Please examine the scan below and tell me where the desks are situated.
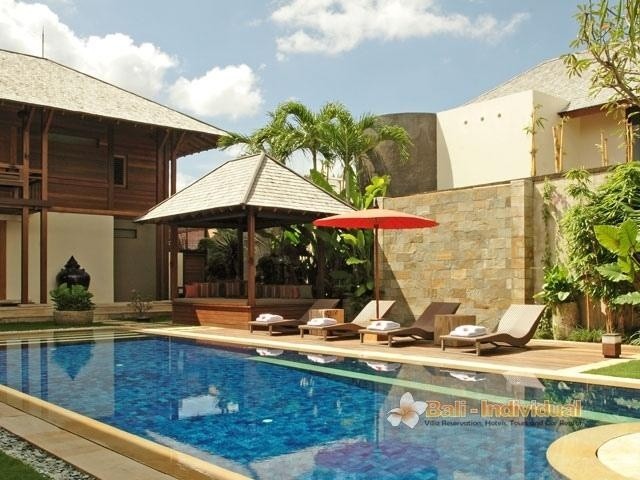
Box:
[546,418,640,480]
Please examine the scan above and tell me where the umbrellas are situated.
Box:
[313,206,440,320]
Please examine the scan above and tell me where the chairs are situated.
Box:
[247,296,547,354]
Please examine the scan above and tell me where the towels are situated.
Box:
[253,348,284,358]
[305,353,337,364]
[447,371,489,384]
[363,359,402,373]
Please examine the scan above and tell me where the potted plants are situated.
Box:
[536,263,579,340]
[558,167,640,360]
[48,283,96,324]
[125,288,154,322]
